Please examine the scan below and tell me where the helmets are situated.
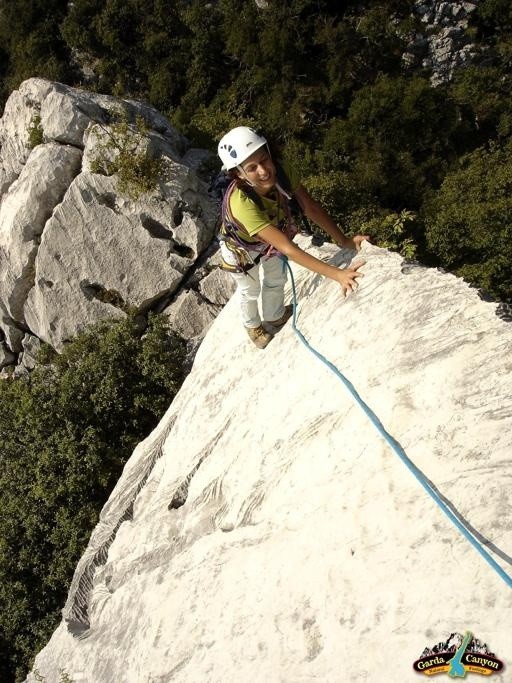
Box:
[218,126,267,172]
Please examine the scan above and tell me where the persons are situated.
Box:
[215,126,372,350]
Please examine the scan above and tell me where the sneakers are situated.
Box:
[246,323,273,349]
[267,305,293,328]
